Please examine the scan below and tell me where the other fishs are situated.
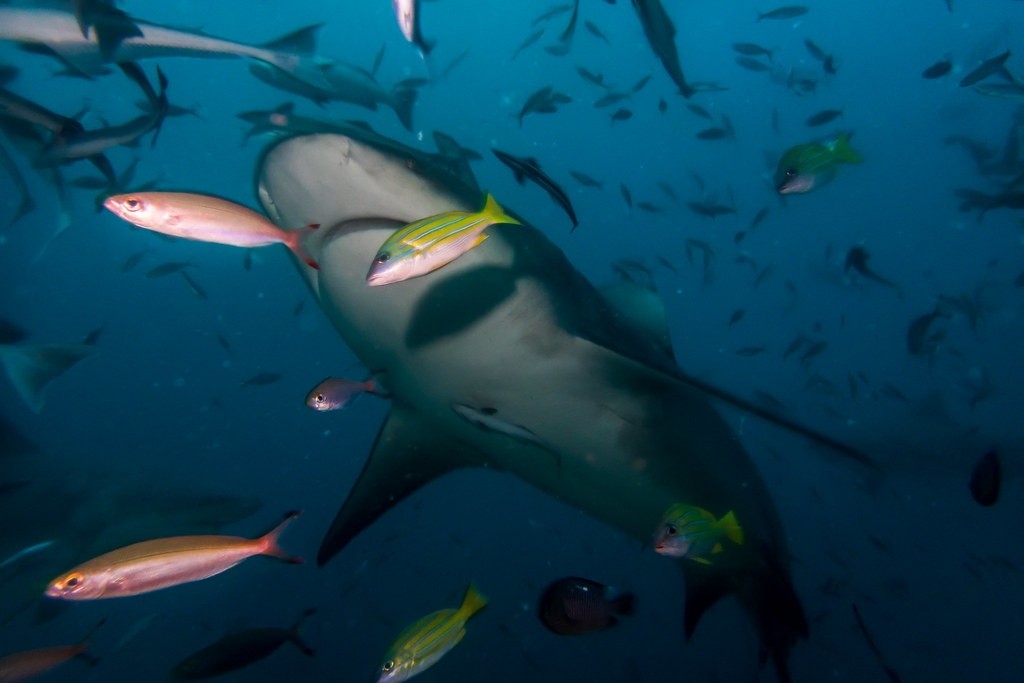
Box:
[0,0,1023,682]
